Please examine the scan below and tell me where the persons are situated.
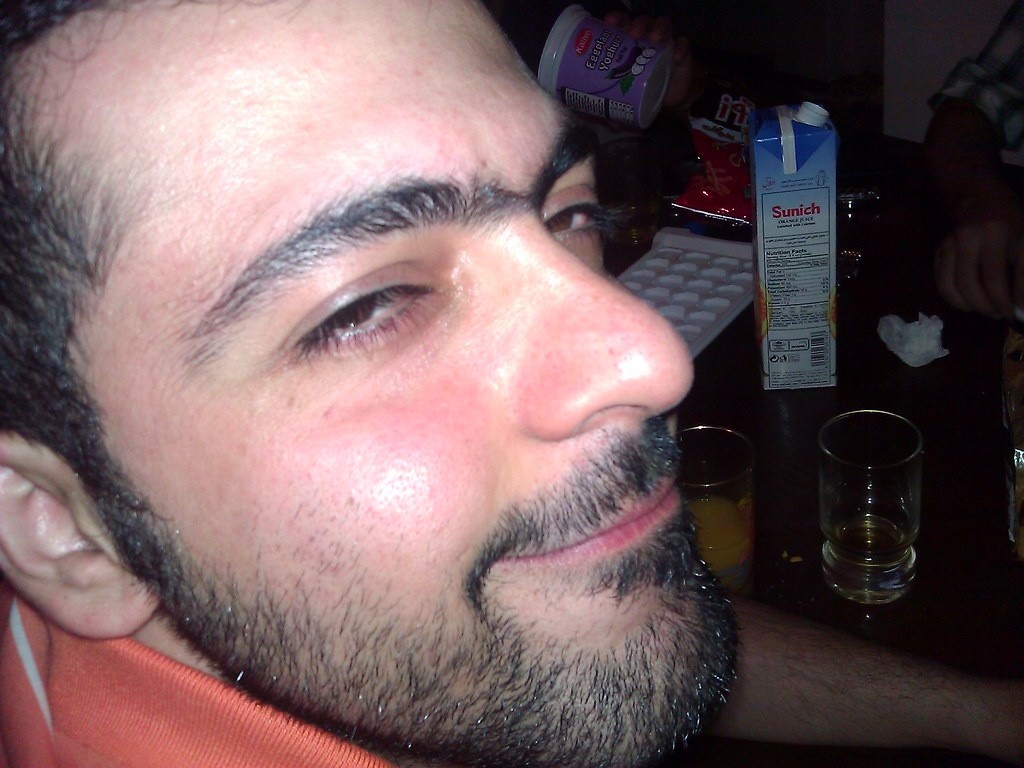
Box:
[483,0,707,131]
[920,0,1024,319]
[0,0,1024,768]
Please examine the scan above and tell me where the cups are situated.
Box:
[676,426,756,590]
[818,410,923,603]
[537,5,670,128]
[596,136,662,245]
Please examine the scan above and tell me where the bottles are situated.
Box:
[1002,304,1024,561]
